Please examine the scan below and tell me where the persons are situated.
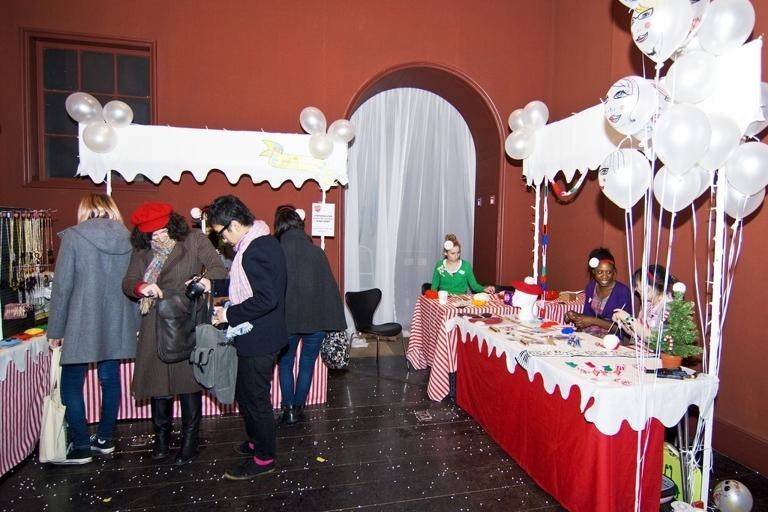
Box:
[47,191,142,465]
[273,206,347,425]
[612,265,673,353]
[430,234,496,296]
[565,248,633,341]
[184,194,291,480]
[121,199,216,466]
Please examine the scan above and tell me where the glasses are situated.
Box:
[216,226,229,238]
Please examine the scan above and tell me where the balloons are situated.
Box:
[103,99,133,129]
[603,76,658,137]
[724,179,766,221]
[65,92,104,125]
[699,0,755,56]
[299,106,326,136]
[654,165,701,213]
[744,82,768,137]
[327,119,355,143]
[507,108,524,131]
[309,133,334,160]
[504,128,536,160]
[82,121,117,153]
[713,479,753,512]
[697,112,741,173]
[724,142,768,195]
[631,0,692,64]
[522,100,550,130]
[652,103,712,175]
[598,147,652,210]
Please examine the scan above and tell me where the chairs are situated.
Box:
[495,283,516,294]
[420,281,434,296]
[342,287,410,384]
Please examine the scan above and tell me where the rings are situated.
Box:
[147,290,153,296]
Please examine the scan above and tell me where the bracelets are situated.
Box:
[624,315,635,325]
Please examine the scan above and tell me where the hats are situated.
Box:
[132,202,172,232]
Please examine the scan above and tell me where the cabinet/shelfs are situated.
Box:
[0,314,328,480]
[414,292,720,512]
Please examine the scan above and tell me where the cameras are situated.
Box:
[185,279,206,299]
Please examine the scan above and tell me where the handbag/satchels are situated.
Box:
[39,346,67,463]
[157,230,210,364]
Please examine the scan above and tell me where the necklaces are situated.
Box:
[0,210,54,294]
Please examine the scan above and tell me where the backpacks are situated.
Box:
[158,290,210,364]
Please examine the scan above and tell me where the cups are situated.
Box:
[438,291,447,305]
[602,334,619,350]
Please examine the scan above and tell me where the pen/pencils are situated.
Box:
[490,327,497,332]
[520,340,526,345]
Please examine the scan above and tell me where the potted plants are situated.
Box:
[640,296,702,371]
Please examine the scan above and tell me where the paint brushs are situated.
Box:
[646,366,701,379]
[608,304,627,333]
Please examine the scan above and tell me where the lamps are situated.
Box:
[489,195,497,206]
[476,197,483,209]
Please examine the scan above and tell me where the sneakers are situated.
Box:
[225,457,274,480]
[55,442,92,464]
[90,434,115,454]
[235,441,255,456]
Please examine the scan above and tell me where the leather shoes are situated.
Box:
[151,443,171,460]
[175,442,198,466]
[283,404,299,423]
